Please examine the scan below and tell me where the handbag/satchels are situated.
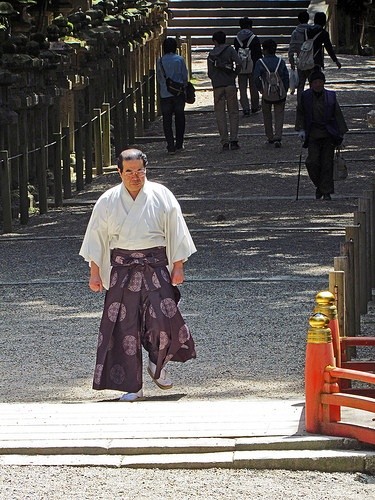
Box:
[333,146,348,180]
[289,66,298,95]
[166,78,186,98]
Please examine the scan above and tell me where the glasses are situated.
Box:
[119,168,148,178]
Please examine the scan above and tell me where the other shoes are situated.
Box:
[168,146,182,155]
[223,144,229,150]
[231,141,240,150]
[251,108,262,115]
[268,140,281,148]
[243,112,250,117]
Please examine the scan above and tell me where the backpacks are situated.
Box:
[236,34,256,74]
[258,57,287,102]
[297,29,324,71]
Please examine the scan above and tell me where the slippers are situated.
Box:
[147,366,174,390]
[117,397,146,402]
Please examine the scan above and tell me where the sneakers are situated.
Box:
[316,187,323,199]
[324,193,331,201]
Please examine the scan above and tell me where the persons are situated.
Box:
[253,39,290,147]
[207,31,242,148]
[296,72,348,199]
[288,12,311,70]
[79,149,197,401]
[156,39,195,151]
[296,12,342,104]
[234,17,261,116]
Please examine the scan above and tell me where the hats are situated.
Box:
[307,71,326,83]
